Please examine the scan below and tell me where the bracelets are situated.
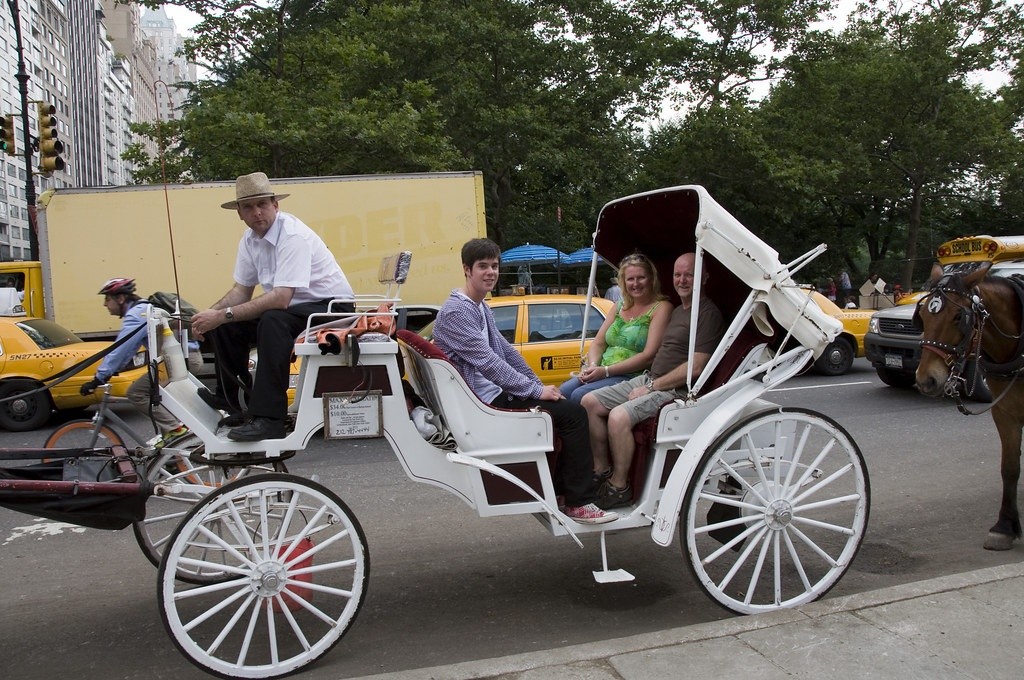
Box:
[605,366,611,379]
[647,380,655,392]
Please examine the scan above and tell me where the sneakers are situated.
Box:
[588,464,615,493]
[154,423,190,449]
[157,465,178,477]
[557,495,566,510]
[594,479,634,510]
[197,387,235,416]
[227,417,286,442]
[564,502,619,525]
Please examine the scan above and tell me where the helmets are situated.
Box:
[97,278,137,296]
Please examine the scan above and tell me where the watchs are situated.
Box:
[225,306,234,323]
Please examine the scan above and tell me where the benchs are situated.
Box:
[633,326,773,451]
[294,250,414,356]
[395,328,554,458]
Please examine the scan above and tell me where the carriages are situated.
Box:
[0,179,872,680]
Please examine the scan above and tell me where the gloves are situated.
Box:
[79,376,103,396]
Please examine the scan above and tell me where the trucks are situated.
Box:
[863,232,1024,402]
[0,170,485,342]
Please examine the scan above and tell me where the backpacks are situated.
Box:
[130,291,201,326]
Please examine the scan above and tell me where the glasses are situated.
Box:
[621,254,652,273]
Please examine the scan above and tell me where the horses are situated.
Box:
[912,261,1024,551]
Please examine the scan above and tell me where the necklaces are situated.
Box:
[629,301,650,323]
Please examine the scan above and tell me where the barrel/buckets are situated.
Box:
[251,503,313,612]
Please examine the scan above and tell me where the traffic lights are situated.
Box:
[38,102,64,171]
[0,115,15,155]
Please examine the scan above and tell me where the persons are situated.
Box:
[558,253,727,511]
[870,273,878,284]
[80,277,203,449]
[825,278,836,304]
[192,172,356,442]
[837,272,851,309]
[7,278,16,287]
[812,279,824,294]
[432,238,618,525]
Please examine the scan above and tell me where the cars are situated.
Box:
[896,275,932,305]
[796,287,879,376]
[397,295,616,391]
[0,317,170,432]
[242,304,442,416]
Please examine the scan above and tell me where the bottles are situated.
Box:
[161,327,188,381]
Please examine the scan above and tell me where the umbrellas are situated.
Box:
[499,242,569,294]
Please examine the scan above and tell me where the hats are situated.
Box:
[826,278,833,282]
[609,277,618,284]
[220,172,290,210]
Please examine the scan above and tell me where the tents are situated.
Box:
[553,248,603,293]
